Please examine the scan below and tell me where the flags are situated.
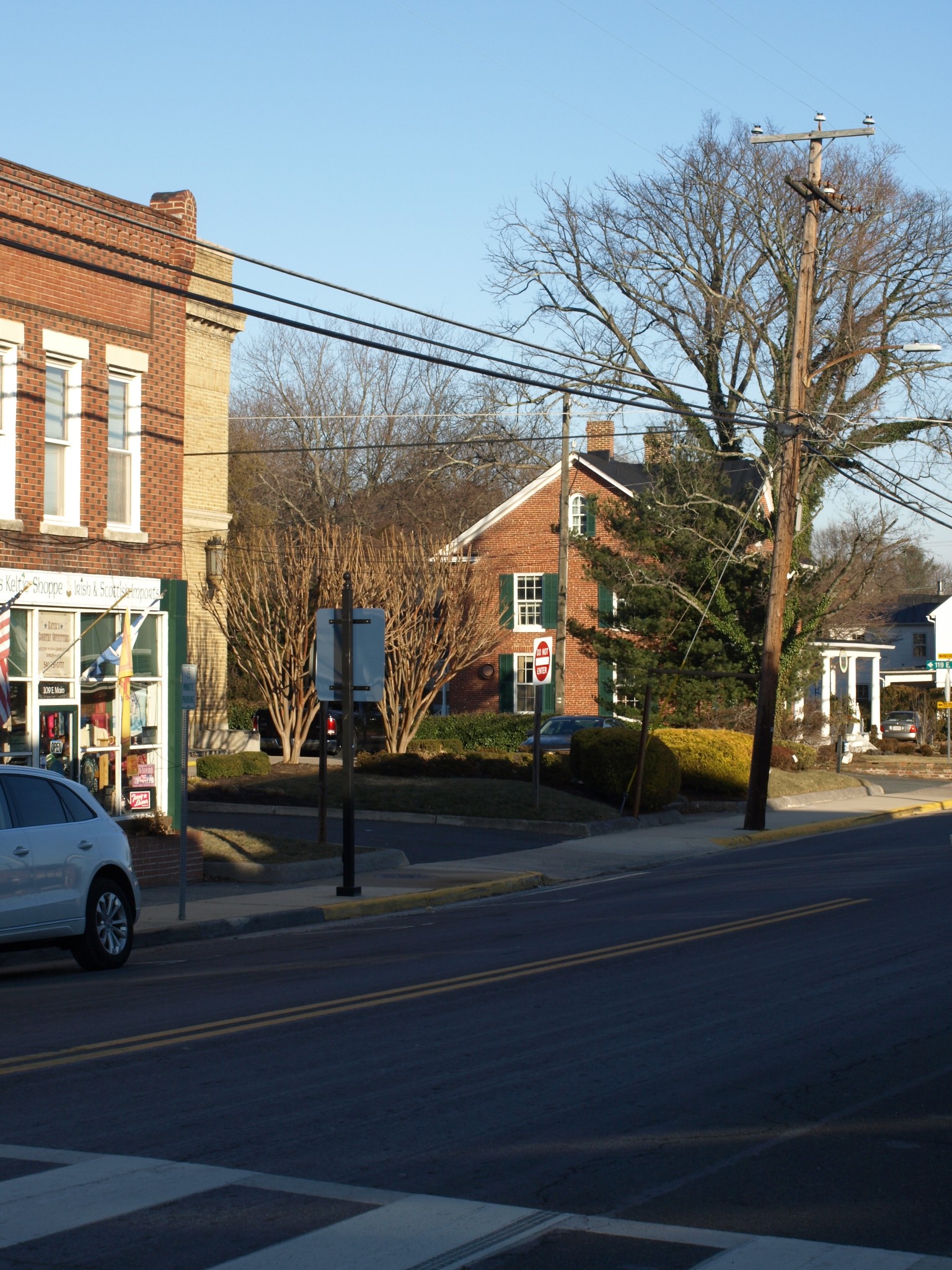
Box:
[0,589,150,754]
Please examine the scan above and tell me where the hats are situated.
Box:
[81,717,90,728]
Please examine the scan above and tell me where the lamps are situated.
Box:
[207,534,225,592]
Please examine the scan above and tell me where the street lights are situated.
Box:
[740,339,944,831]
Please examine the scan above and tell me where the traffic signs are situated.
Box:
[937,702,952,709]
[926,660,952,669]
[938,654,952,659]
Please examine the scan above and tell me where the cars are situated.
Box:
[880,710,923,746]
[0,764,142,972]
[253,685,388,756]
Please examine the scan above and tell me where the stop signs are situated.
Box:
[535,641,550,682]
